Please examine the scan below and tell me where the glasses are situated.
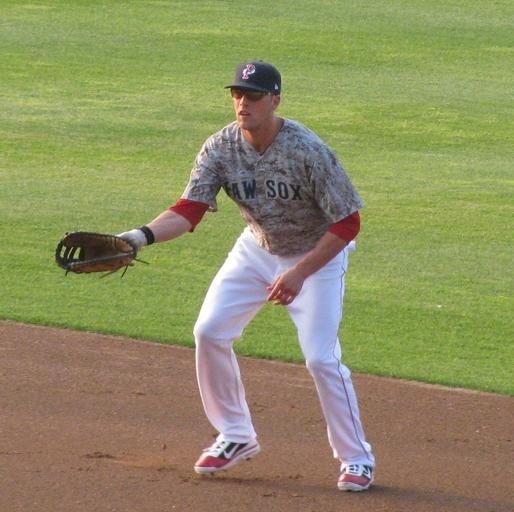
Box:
[231,86,270,101]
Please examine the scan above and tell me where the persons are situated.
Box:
[114,57,377,493]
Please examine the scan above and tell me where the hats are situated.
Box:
[224,60,282,95]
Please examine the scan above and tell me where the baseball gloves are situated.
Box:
[56,232,136,274]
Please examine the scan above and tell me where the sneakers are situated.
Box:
[337,463,374,492]
[193,436,261,476]
